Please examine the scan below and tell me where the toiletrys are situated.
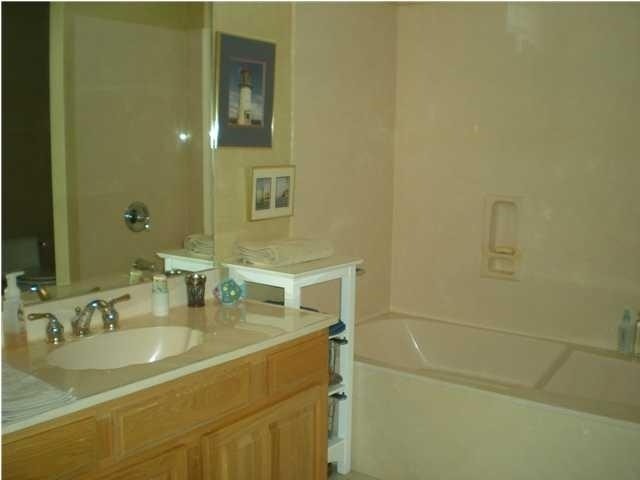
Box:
[3,270,29,349]
[149,271,171,318]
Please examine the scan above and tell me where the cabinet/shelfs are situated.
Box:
[221,251,364,474]
[155,249,213,276]
[1,327,329,480]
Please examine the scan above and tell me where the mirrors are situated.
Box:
[2,1,213,312]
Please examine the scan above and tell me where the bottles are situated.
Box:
[151,274,169,316]
[618,310,640,356]
[129,270,143,285]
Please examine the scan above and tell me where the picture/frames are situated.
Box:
[215,29,277,149]
[249,164,296,221]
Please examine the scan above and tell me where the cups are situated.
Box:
[185,277,206,306]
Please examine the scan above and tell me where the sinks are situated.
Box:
[44,321,209,372]
[21,284,98,304]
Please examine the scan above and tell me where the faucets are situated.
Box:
[29,283,49,299]
[69,296,119,336]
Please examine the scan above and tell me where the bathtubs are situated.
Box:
[353,311,639,479]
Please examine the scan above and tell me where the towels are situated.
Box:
[240,239,334,267]
[184,234,214,260]
[1,362,78,421]
